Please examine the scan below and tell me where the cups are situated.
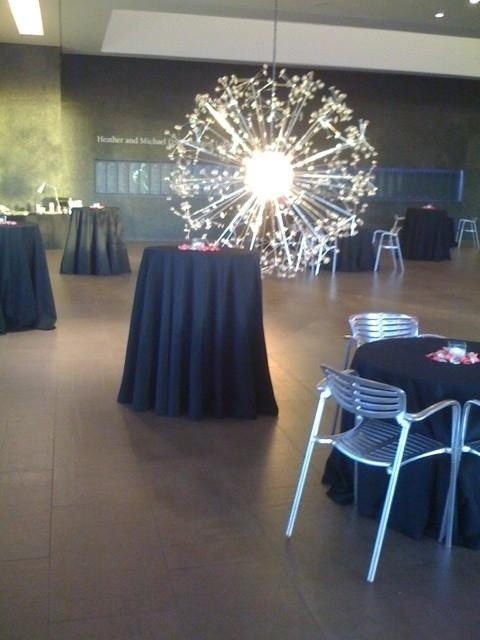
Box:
[1,215,6,224]
[448,341,467,362]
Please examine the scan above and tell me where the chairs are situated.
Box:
[437,399,480,546]
[329,311,446,438]
[286,363,460,583]
[456,216,479,250]
[370,216,406,275]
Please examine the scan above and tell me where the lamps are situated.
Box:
[37,183,62,212]
[162,0,378,280]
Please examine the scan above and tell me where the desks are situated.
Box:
[118,246,278,420]
[0,211,72,250]
[397,206,456,263]
[0,219,57,335]
[321,338,480,552]
[306,222,379,272]
[60,208,131,276]
